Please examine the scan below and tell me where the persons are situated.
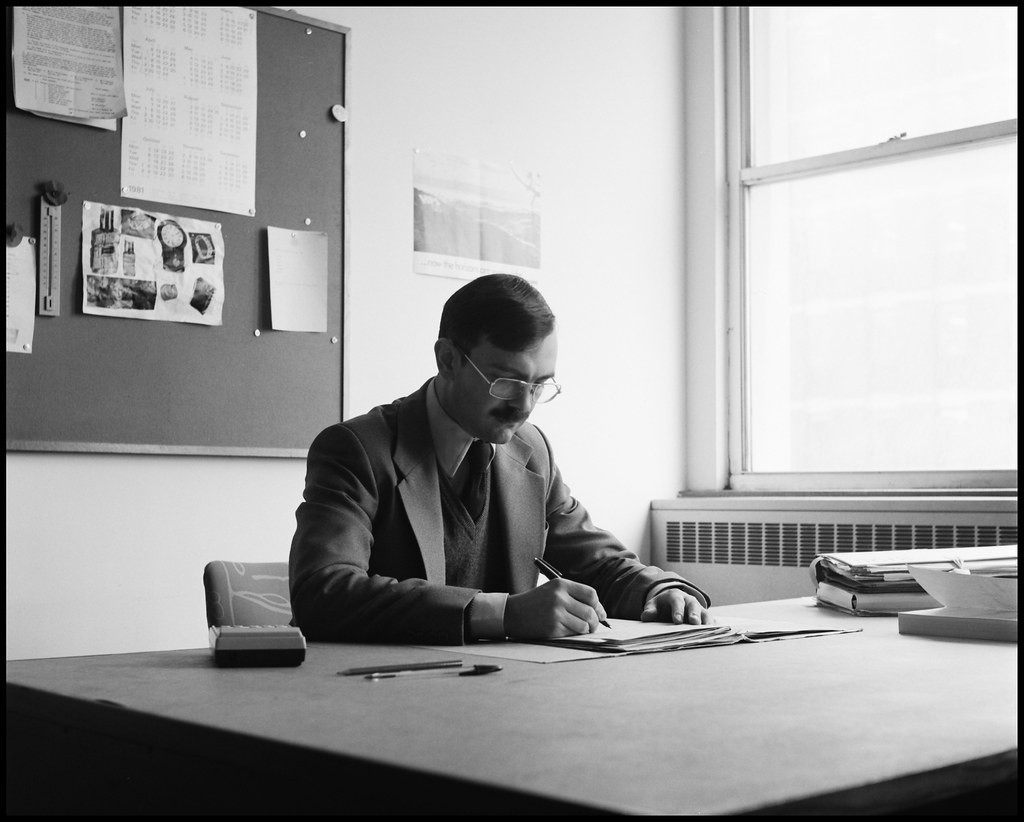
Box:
[288,274,712,641]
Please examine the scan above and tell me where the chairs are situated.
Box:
[205,561,294,628]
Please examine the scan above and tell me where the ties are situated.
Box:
[459,440,494,525]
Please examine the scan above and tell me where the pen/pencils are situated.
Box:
[335,659,503,680]
[532,558,612,630]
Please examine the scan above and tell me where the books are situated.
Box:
[897,563,1017,644]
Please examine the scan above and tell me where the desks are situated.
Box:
[7,595,1022,820]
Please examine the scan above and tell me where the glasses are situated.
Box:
[462,352,563,406]
